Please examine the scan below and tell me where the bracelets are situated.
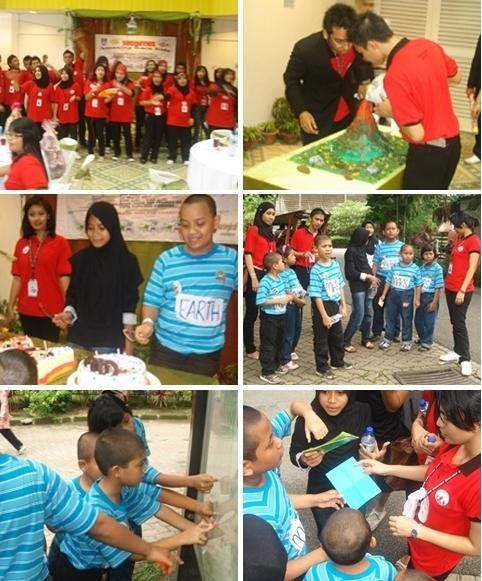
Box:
[142,317,155,326]
[458,289,467,296]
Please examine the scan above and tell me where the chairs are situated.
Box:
[462,154,480,164]
[315,369,336,380]
[364,505,388,532]
[439,350,461,362]
[330,362,352,370]
[259,374,281,384]
[284,361,299,370]
[289,351,298,360]
[459,359,473,376]
[365,335,431,352]
[77,140,193,166]
[342,344,357,353]
[246,350,262,361]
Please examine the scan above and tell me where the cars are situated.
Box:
[207,129,238,155]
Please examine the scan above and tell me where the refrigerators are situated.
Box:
[273,365,289,375]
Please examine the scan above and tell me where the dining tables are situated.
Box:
[243,97,301,152]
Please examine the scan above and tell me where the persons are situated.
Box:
[344,220,446,355]
[245,199,279,362]
[276,245,308,373]
[282,2,377,147]
[289,207,329,290]
[348,9,462,190]
[1,453,186,581]
[1,349,39,386]
[43,391,223,581]
[350,1,376,82]
[255,253,293,385]
[437,210,480,379]
[306,232,353,382]
[0,28,237,165]
[134,193,238,378]
[1,390,27,457]
[7,197,73,344]
[1,119,51,190]
[463,33,480,166]
[242,390,480,581]
[53,202,145,358]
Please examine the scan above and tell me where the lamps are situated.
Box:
[60,138,78,152]
[70,154,96,189]
[146,167,189,190]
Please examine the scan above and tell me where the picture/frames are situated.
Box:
[1,330,222,385]
[243,123,408,189]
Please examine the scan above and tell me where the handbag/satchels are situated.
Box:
[458,289,467,296]
[142,317,155,326]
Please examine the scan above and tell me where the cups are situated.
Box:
[6,131,23,139]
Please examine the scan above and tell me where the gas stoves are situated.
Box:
[1,335,151,386]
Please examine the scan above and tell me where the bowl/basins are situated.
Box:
[333,50,347,77]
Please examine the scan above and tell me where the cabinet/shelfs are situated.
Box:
[418,396,430,415]
[329,312,342,327]
[360,427,376,454]
[280,289,309,300]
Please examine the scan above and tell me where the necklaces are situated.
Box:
[333,50,347,77]
